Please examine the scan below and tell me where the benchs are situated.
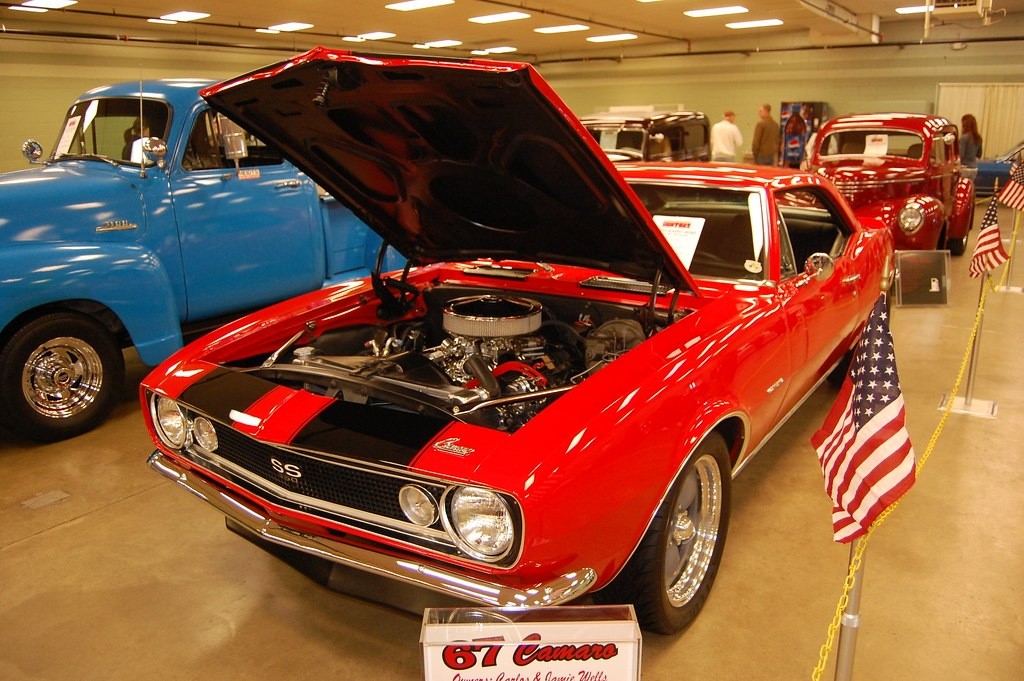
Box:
[652,209,840,272]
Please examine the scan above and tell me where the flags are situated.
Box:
[997,154,1024,211]
[809,290,916,544]
[968,193,1011,279]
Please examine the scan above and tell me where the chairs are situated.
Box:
[842,142,864,153]
[908,144,923,158]
[648,135,671,154]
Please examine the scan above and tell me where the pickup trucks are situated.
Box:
[0,80,412,443]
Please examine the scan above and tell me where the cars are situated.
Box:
[974,140,1024,200]
[579,111,711,172]
[805,113,975,263]
[138,44,896,635]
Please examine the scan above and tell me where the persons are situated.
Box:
[711,111,742,162]
[960,115,983,182]
[752,105,778,165]
[122,117,150,160]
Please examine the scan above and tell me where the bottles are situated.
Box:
[784,104,807,170]
[802,106,813,139]
